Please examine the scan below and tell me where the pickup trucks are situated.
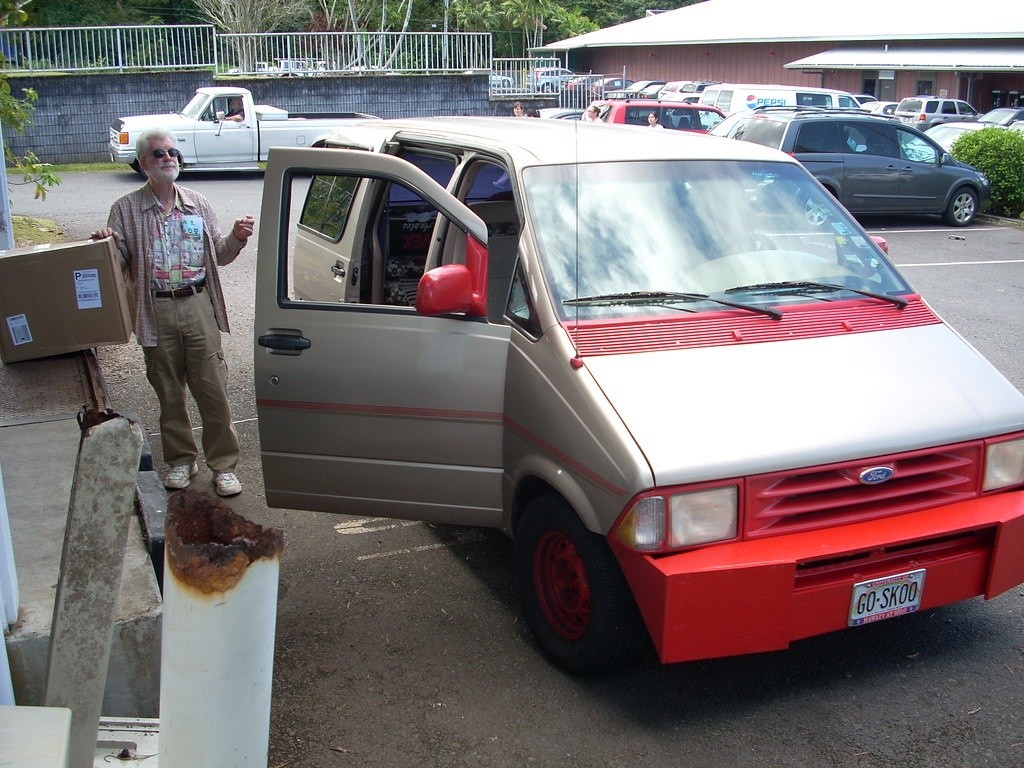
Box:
[105,86,386,177]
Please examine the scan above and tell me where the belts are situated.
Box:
[151,280,207,297]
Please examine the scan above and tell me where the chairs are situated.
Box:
[382,201,530,325]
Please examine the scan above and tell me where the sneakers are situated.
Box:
[164,460,197,489]
[211,472,242,496]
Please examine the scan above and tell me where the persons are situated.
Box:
[694,180,777,260]
[588,106,603,123]
[225,97,244,122]
[524,109,540,117]
[513,102,524,117]
[648,112,663,128]
[90,129,255,496]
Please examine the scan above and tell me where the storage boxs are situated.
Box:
[0,235,133,364]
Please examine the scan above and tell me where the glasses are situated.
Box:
[144,147,181,156]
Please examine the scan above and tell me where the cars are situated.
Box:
[457,63,1024,226]
[253,110,1024,685]
